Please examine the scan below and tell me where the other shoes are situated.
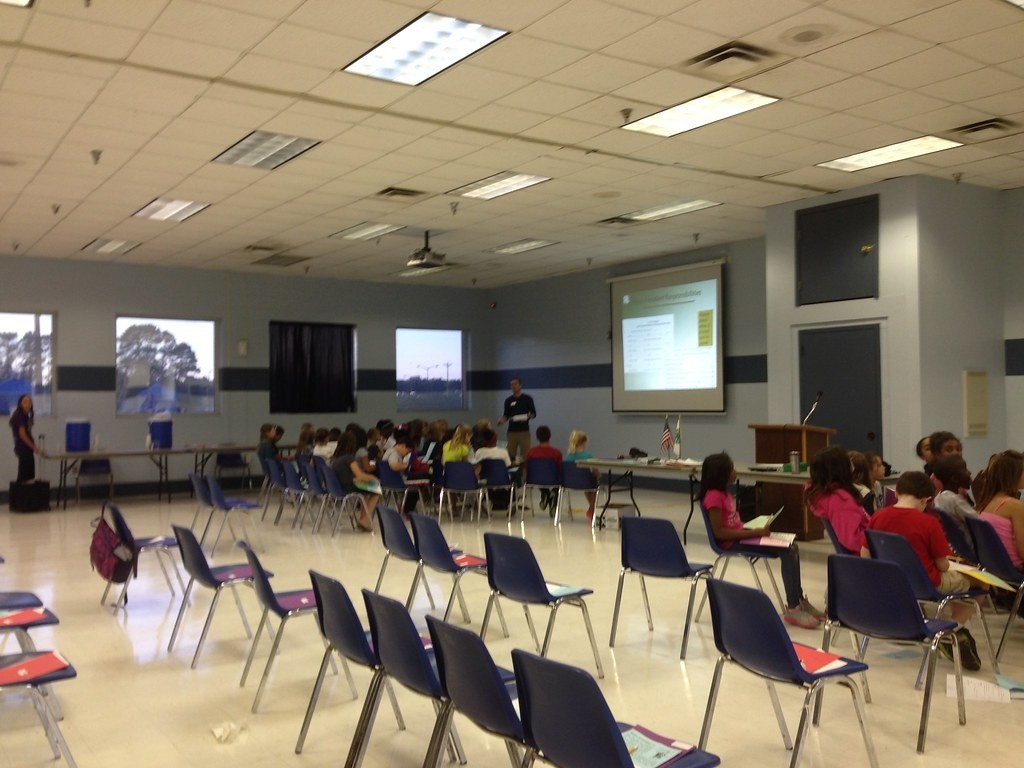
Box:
[586,510,593,519]
[539,493,550,510]
[549,498,558,517]
[358,522,372,532]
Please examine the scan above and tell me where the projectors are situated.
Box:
[407,252,446,267]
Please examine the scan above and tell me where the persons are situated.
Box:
[9,395,42,485]
[804,443,885,559]
[693,453,825,628]
[565,430,601,519]
[914,431,979,556]
[976,449,1024,562]
[861,470,989,671]
[258,419,519,532]
[525,426,563,517]
[497,379,536,459]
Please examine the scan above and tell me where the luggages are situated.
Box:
[10,434,52,514]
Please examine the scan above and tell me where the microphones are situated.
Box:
[815,390,823,402]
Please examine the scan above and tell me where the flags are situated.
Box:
[673,420,680,456]
[661,421,673,453]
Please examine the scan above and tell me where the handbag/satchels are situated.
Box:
[88,519,132,585]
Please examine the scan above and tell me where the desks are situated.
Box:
[574,458,906,546]
[34,443,304,511]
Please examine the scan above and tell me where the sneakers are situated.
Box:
[783,605,821,627]
[798,596,826,620]
[955,628,982,671]
[937,638,954,660]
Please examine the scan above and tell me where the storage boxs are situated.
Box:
[148,415,174,449]
[594,504,635,530]
[66,419,91,451]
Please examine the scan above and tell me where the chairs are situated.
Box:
[0,441,1024,768]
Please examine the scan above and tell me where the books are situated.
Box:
[740,532,797,548]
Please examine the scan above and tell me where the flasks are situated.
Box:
[789,451,801,475]
